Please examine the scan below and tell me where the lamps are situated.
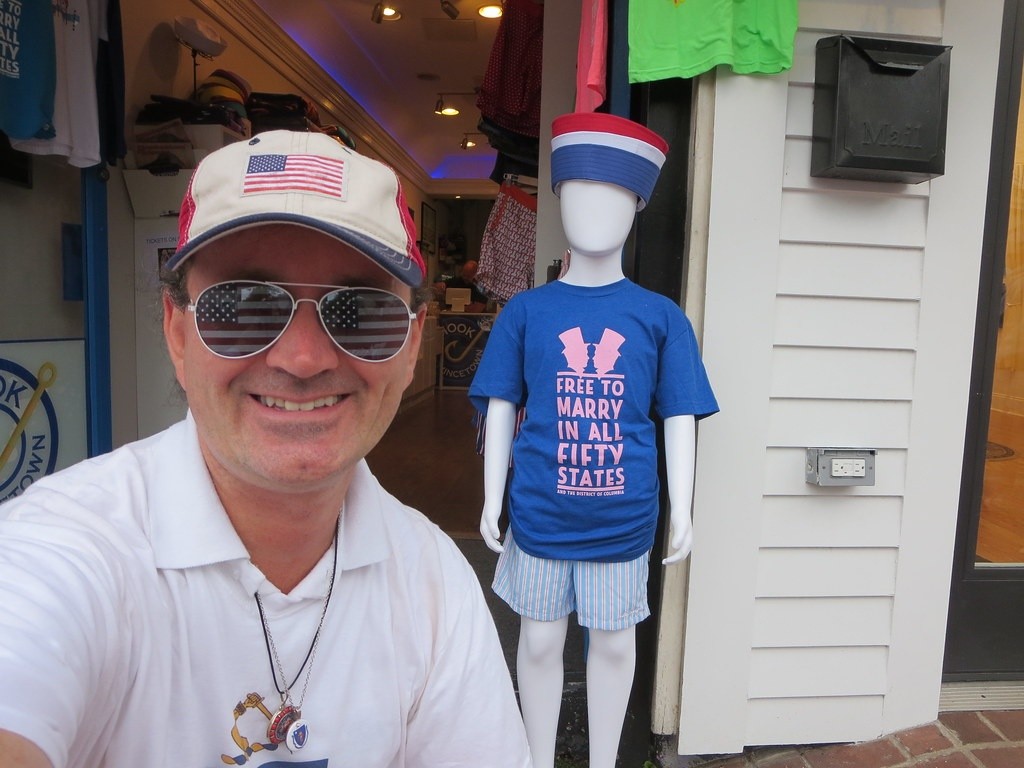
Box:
[371,0,385,25]
[439,0,458,20]
[434,95,444,115]
[462,133,470,149]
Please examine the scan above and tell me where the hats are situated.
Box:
[197,67,253,120]
[173,16,227,57]
[162,128,429,289]
[551,112,671,212]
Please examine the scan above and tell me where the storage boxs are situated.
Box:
[120,96,253,216]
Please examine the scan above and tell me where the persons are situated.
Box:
[0,128,536,768]
[431,258,490,314]
[467,114,720,768]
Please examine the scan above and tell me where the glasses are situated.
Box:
[184,278,419,364]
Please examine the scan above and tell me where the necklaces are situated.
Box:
[235,517,342,759]
